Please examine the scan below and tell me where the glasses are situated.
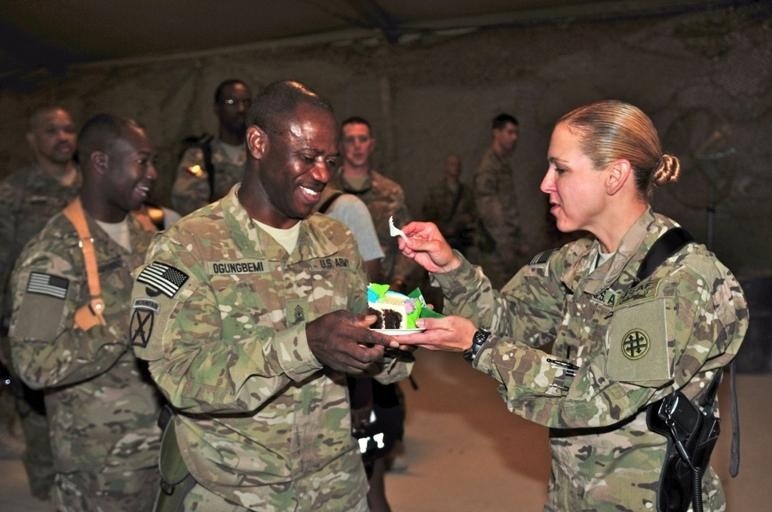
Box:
[221,96,251,107]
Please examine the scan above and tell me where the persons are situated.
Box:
[1,79,416,512]
[386,98,751,512]
[472,113,530,289]
[419,154,476,260]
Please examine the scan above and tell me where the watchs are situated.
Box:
[463,329,491,361]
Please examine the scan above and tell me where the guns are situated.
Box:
[658,390,721,476]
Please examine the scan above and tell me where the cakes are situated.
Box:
[367,302,405,329]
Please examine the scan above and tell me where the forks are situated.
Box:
[388,215,410,242]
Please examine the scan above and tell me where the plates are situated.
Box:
[365,326,425,338]
